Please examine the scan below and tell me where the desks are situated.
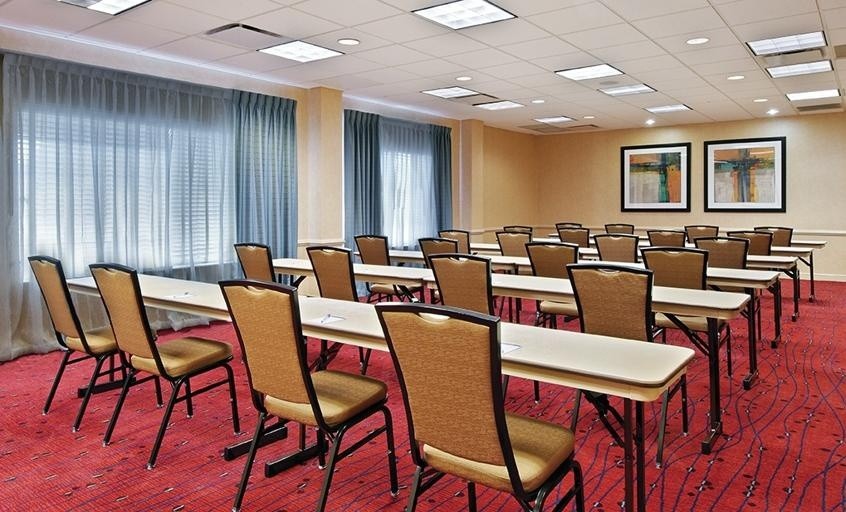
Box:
[63,271,696,512]
[353,249,781,391]
[272,257,752,456]
[533,237,813,300]
[547,233,829,302]
[461,242,803,320]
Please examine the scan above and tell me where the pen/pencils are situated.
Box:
[321,312,330,324]
[173,292,188,299]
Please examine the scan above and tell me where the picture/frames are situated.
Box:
[620,142,691,213]
[704,136,787,213]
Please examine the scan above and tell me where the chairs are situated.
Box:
[428,254,540,404]
[496,231,532,256]
[418,237,460,305]
[726,231,773,256]
[27,255,158,433]
[593,234,642,263]
[504,225,533,231]
[88,263,241,468]
[525,242,580,329]
[685,225,719,244]
[640,247,734,380]
[374,302,585,512]
[754,226,794,247]
[555,222,582,230]
[566,263,689,467]
[438,229,471,254]
[693,236,762,342]
[558,227,590,248]
[647,230,686,248]
[605,223,634,234]
[234,242,276,365]
[354,235,427,305]
[218,278,399,512]
[306,246,364,371]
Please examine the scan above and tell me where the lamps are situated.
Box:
[338,38,361,47]
[642,103,694,115]
[58,1,155,17]
[410,0,519,31]
[764,59,835,80]
[554,63,625,82]
[785,88,842,102]
[455,76,473,82]
[472,99,526,111]
[257,39,346,63]
[421,86,481,99]
[745,30,829,57]
[686,36,710,46]
[597,82,658,97]
